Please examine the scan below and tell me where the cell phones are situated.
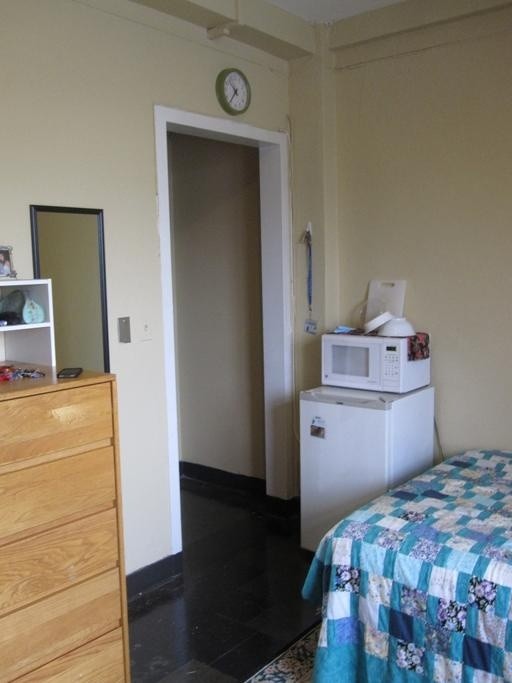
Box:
[57,368,82,379]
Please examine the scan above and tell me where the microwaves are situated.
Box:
[321,334,431,393]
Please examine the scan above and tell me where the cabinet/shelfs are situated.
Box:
[1,279,57,364]
[0,360,129,681]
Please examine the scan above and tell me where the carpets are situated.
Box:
[245,621,321,682]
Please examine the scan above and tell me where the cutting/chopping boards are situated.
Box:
[365,279,406,322]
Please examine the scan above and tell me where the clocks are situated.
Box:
[216,68,251,114]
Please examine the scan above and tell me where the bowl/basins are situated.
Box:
[378,318,416,336]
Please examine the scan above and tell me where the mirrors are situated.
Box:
[26,204,111,372]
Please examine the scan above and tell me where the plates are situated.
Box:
[363,311,391,336]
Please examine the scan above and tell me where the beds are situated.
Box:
[301,446,510,676]
